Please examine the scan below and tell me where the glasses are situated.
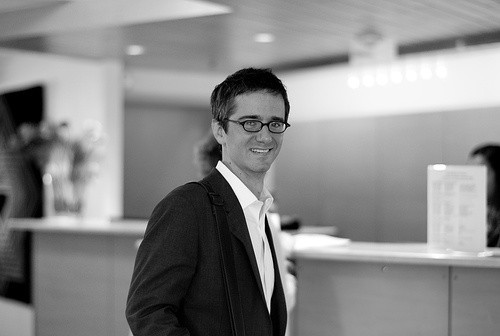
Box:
[226,119,290,134]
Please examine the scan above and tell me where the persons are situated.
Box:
[126,68,291,336]
[195,129,221,180]
[467,144,500,248]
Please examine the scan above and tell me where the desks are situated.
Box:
[10,220,499,336]
